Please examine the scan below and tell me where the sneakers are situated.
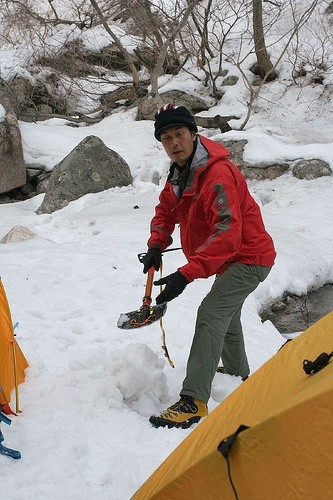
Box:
[217,367,248,382]
[149,396,208,429]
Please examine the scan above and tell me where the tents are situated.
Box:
[125,310,333,500]
[0,277,30,405]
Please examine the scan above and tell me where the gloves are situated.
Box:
[142,248,162,274]
[153,271,189,305]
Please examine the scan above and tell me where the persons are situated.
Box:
[141,102,276,431]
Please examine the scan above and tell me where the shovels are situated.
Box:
[116,266,167,331]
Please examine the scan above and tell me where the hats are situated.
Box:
[154,103,198,142]
